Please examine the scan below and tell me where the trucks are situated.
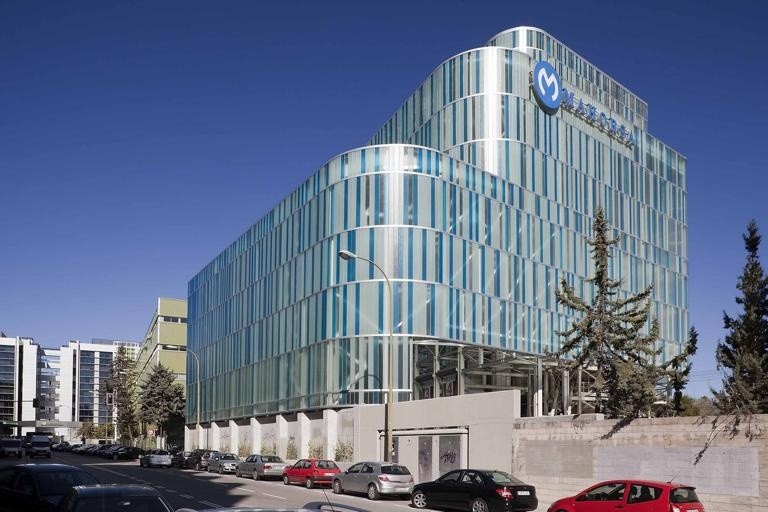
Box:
[0,431,53,460]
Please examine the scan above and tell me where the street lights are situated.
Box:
[166,344,202,426]
[118,371,144,448]
[89,390,108,445]
[335,250,397,463]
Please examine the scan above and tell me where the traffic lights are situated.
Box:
[32,398,40,408]
[105,392,114,404]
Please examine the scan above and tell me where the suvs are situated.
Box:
[176,500,374,512]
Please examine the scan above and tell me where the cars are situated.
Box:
[135,446,241,476]
[0,464,100,511]
[330,461,415,501]
[234,453,290,482]
[57,484,199,512]
[547,478,706,512]
[409,468,538,512]
[282,459,342,489]
[52,440,146,459]
